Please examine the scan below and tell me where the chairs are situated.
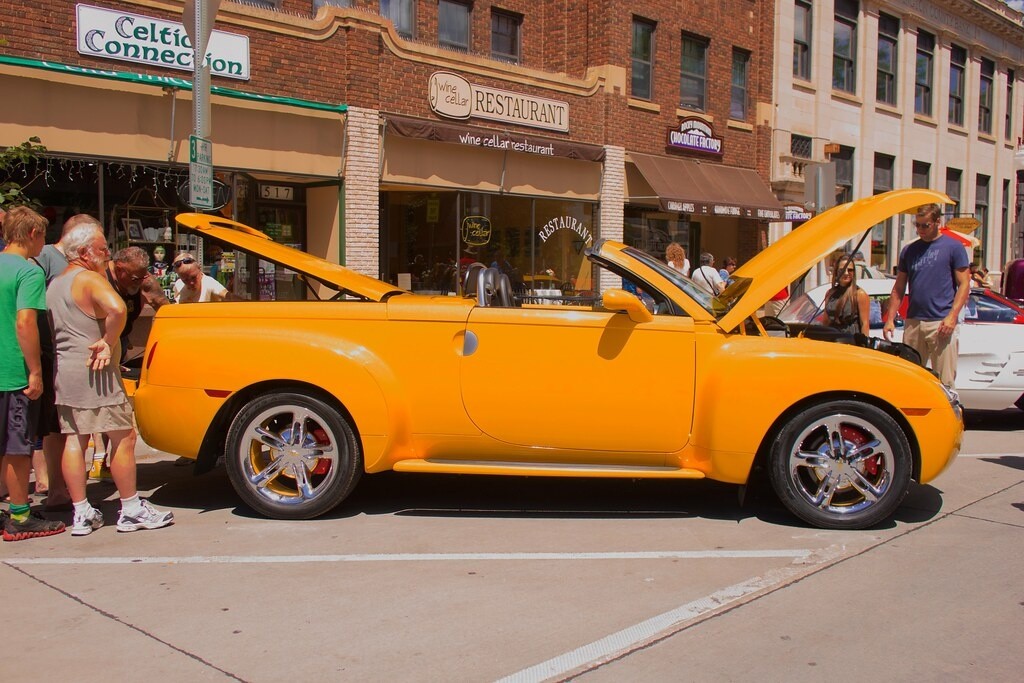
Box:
[499,274,515,307]
[477,268,503,307]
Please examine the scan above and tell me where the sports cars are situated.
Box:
[119,188,967,532]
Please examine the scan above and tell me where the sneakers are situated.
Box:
[3,511,66,541]
[0,508,44,535]
[71,506,104,536]
[117,499,174,531]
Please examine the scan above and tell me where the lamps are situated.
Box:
[573,241,585,254]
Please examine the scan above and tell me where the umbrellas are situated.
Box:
[906,227,981,249]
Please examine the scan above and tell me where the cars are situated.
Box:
[764,277,1024,416]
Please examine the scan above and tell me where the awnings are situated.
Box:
[627,152,785,220]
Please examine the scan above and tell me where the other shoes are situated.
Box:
[88,459,113,480]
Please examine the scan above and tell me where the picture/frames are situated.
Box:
[121,218,146,242]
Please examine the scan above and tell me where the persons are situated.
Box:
[623,243,790,318]
[406,245,513,293]
[0,206,246,541]
[969,261,993,289]
[824,255,869,338]
[883,203,970,389]
[147,246,173,277]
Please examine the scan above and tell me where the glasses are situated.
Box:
[730,263,735,266]
[175,257,195,268]
[838,268,853,272]
[914,221,929,228]
